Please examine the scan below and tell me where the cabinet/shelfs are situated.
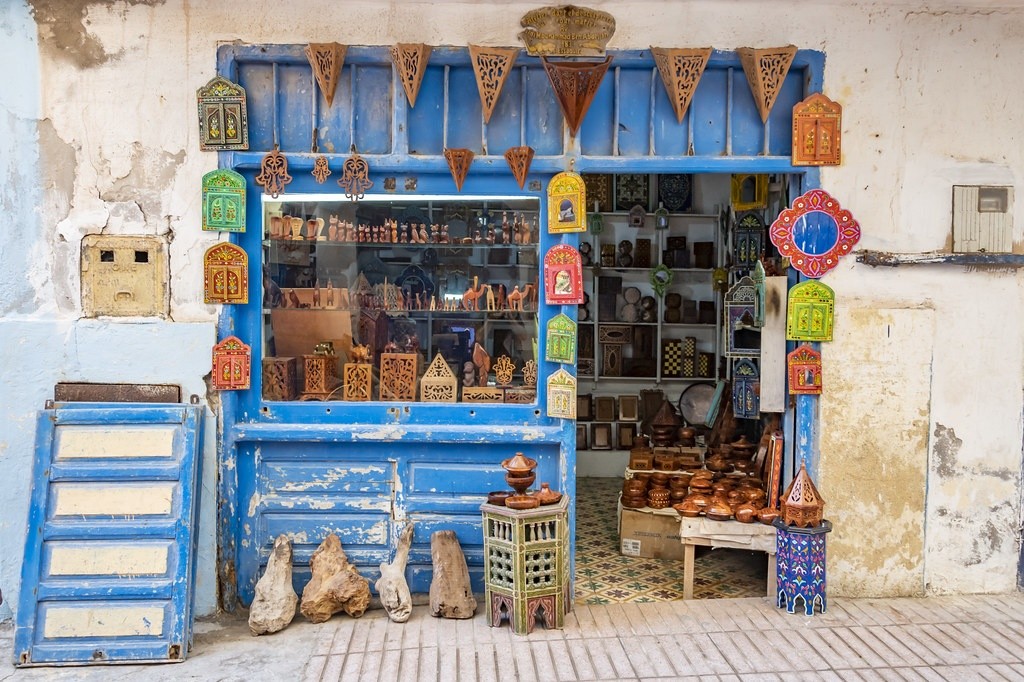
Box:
[262,199,726,478]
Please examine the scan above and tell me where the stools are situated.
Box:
[772,516,834,616]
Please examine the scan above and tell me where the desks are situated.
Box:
[625,459,778,600]
[479,495,571,636]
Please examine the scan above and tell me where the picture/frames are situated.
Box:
[767,432,784,509]
[615,423,636,450]
[577,395,593,422]
[595,397,614,422]
[590,423,612,450]
[618,396,638,422]
[658,174,693,214]
[615,174,649,212]
[580,174,613,213]
[577,424,587,450]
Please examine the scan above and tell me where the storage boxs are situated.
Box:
[603,344,622,376]
[599,325,633,344]
[619,509,687,562]
[632,326,653,361]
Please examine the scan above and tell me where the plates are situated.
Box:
[504,497,540,508]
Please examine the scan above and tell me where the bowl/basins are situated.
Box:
[488,491,513,505]
[621,458,781,525]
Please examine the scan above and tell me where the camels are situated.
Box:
[507,284,533,312]
[463,284,488,311]
